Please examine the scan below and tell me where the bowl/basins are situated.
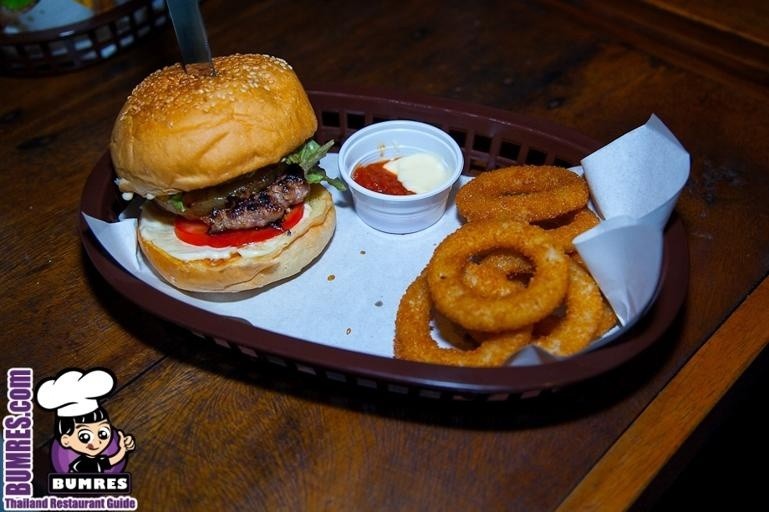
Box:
[337,120,466,235]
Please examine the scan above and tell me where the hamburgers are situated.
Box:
[111,55,346,294]
